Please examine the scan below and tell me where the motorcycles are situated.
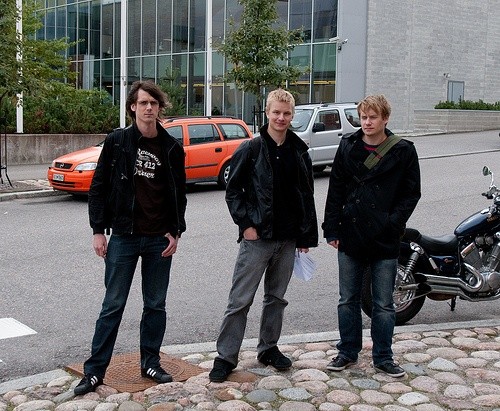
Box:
[361,165,499,326]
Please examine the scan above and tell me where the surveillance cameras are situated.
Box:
[329,36,340,43]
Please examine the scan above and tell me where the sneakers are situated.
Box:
[375,359,405,377]
[259,346,293,369]
[326,355,359,371]
[74,373,103,395]
[141,364,173,383]
[208,360,232,382]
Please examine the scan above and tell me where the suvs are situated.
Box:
[47,115,252,198]
[248,101,363,172]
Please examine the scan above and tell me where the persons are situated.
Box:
[211,106,221,116]
[321,94,421,377]
[74,80,187,396]
[208,88,318,383]
